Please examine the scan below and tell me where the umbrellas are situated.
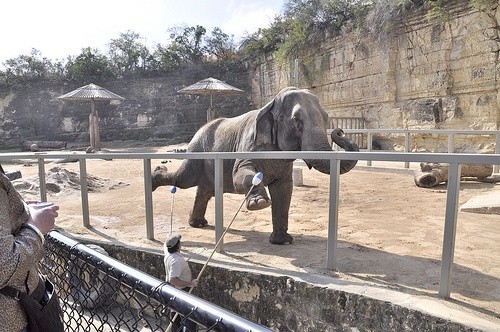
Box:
[57,83,125,113]
[178,77,245,106]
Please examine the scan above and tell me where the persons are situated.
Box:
[164,234,199,332]
[0,165,59,332]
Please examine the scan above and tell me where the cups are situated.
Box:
[30,202,53,210]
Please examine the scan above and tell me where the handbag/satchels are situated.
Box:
[19,272,66,332]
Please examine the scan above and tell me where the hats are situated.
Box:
[166,233,181,248]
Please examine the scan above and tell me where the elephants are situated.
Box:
[151,85,360,245]
